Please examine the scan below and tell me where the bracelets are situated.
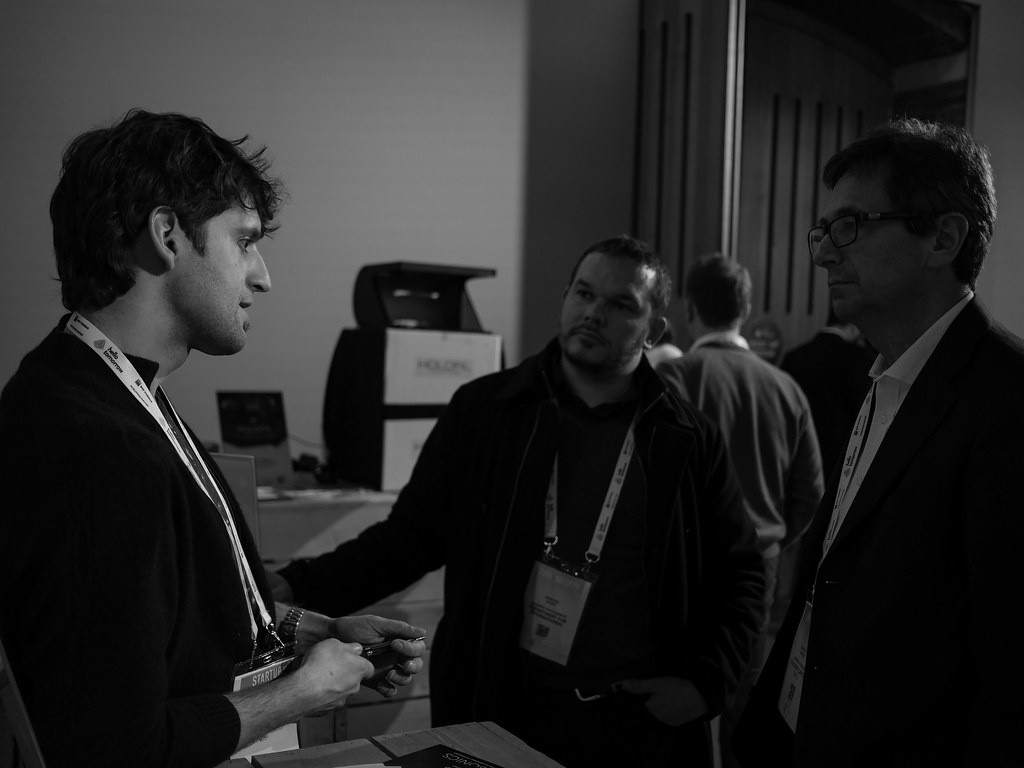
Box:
[283,607,302,646]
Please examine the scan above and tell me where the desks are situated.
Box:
[215,757,255,768]
[251,719,565,768]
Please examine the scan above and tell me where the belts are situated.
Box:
[514,672,622,702]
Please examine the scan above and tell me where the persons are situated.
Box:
[780,309,869,597]
[646,328,683,367]
[266,235,766,768]
[657,254,824,768]
[0,107,425,768]
[733,125,1024,768]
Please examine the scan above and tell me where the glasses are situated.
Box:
[807,210,952,257]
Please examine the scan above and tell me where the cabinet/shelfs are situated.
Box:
[258,484,446,749]
